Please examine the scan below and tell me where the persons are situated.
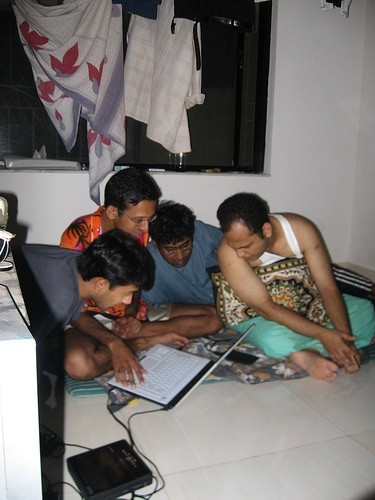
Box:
[47,168,224,389]
[11,226,152,353]
[138,201,224,308]
[216,193,375,381]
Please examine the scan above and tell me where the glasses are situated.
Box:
[158,242,194,256]
[112,202,160,223]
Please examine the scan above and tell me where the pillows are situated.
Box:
[211,258,335,327]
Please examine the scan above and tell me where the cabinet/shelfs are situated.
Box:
[0,242,65,500]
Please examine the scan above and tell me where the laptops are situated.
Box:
[108,323,256,409]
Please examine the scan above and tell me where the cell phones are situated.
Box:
[226,351,259,365]
